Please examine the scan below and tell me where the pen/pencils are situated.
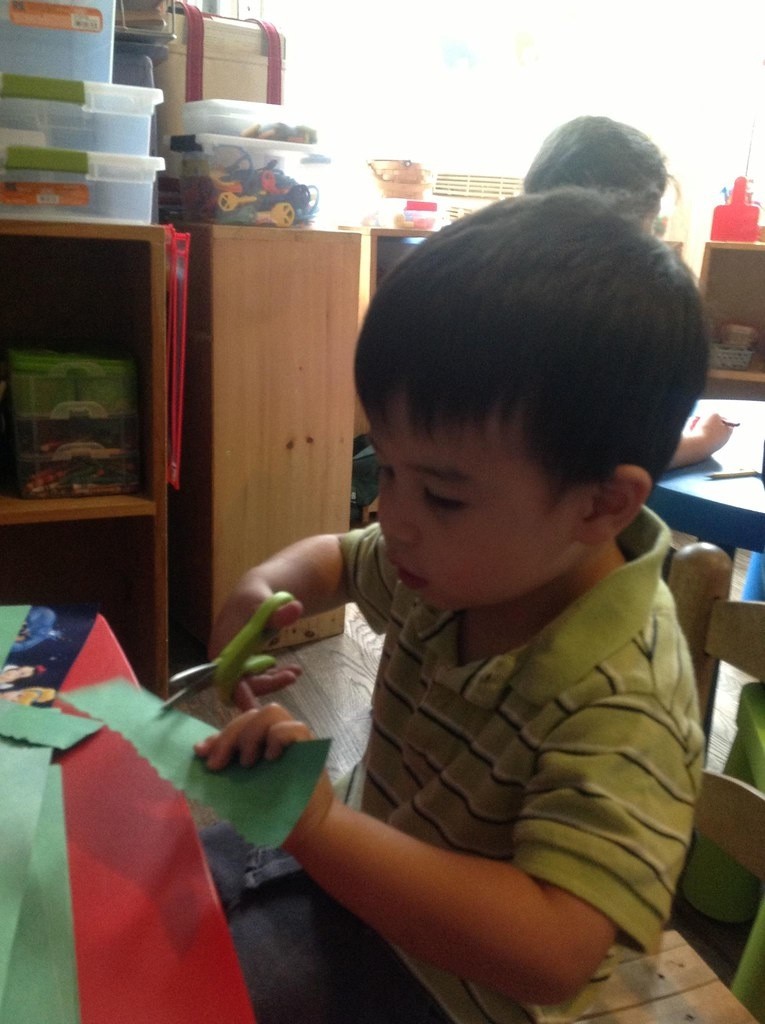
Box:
[696,413,741,428]
[706,469,760,480]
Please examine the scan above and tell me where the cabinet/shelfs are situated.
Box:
[172,225,360,668]
[681,230,764,401]
[0,218,170,702]
[339,223,437,440]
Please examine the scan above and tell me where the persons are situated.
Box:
[194,193,707,1024]
[524,115,730,471]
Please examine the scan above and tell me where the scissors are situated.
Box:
[155,591,296,718]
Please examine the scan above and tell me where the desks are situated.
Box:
[644,399,765,602]
[0,613,258,1023]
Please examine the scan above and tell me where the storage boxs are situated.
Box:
[0,0,331,228]
[6,351,140,499]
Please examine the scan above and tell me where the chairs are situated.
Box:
[665,540,765,878]
